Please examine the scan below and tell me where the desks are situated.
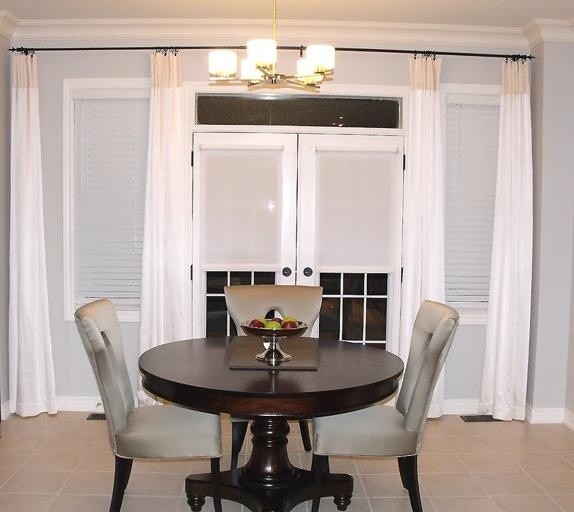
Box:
[138,337,405,512]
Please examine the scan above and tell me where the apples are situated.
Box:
[250,317,298,328]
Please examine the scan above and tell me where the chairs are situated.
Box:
[75,298,223,512]
[223,285,324,469]
[311,300,459,512]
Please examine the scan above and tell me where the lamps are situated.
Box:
[207,2,336,92]
